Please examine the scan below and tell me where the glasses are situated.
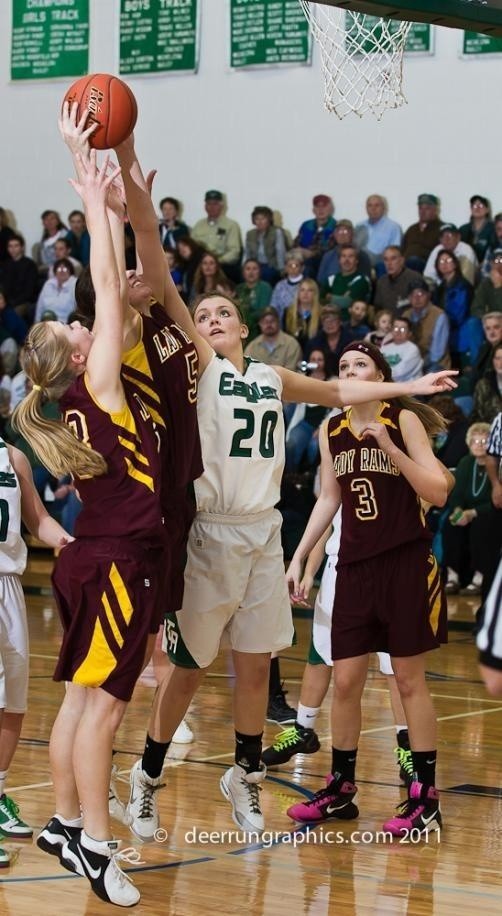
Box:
[56,267,67,272]
[205,192,502,334]
[472,439,487,445]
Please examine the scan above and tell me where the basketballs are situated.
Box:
[62,73,137,150]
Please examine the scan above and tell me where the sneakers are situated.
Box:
[394,744,413,782]
[0,794,33,838]
[383,782,442,836]
[126,758,163,841]
[172,719,193,743]
[60,829,140,907]
[219,759,267,837]
[287,774,358,824]
[0,848,9,867]
[36,813,84,858]
[268,686,297,721]
[261,721,320,766]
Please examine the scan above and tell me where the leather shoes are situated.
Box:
[459,582,481,595]
[444,581,458,594]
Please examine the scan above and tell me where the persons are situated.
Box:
[56,99,203,823]
[1,436,81,867]
[284,341,450,838]
[0,190,501,595]
[469,412,502,698]
[112,131,462,840]
[260,454,456,788]
[10,150,165,903]
